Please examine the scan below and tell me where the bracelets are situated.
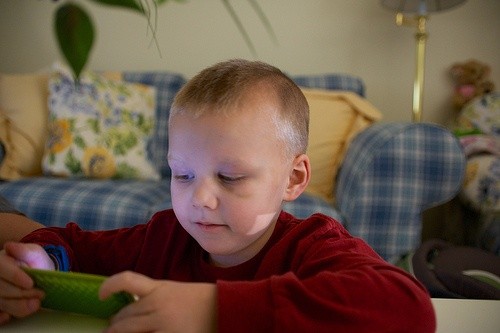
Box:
[45,244,70,273]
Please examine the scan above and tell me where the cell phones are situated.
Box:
[19,267,134,319]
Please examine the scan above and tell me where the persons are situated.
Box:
[0,58,437,333]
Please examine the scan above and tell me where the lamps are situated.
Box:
[381,0,463,120]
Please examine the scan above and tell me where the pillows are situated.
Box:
[41,72,163,183]
[0,69,55,181]
[301,87,383,206]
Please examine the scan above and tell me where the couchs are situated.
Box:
[0,73,465,268]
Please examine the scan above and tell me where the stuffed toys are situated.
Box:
[449,60,499,117]
